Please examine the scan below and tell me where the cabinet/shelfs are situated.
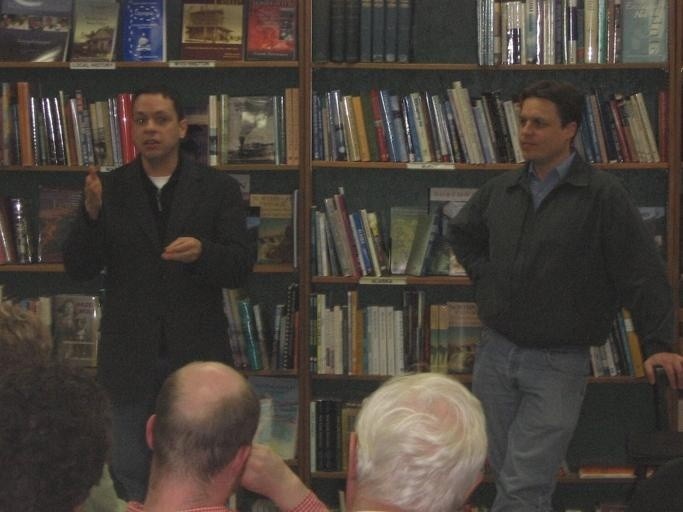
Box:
[305,0,683,511]
[0,0,306,511]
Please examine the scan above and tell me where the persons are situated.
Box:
[63,83,258,503]
[443,78,682,510]
[342,370,493,512]
[0,292,116,510]
[121,356,334,511]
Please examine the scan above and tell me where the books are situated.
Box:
[2,1,300,166]
[1,180,84,265]
[309,398,361,473]
[221,171,298,369]
[556,376,683,480]
[307,177,482,376]
[246,375,300,463]
[312,1,669,165]
[588,307,646,379]
[640,208,666,264]
